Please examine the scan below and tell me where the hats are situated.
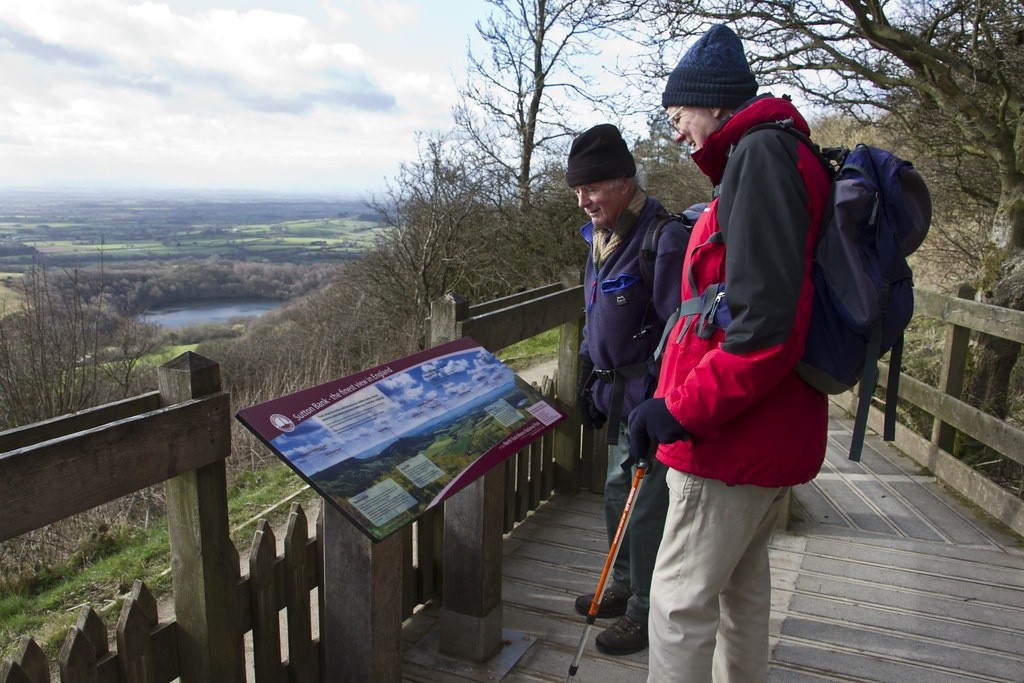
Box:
[564,123,636,186]
[661,24,760,111]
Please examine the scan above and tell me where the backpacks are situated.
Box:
[639,201,711,306]
[718,122,932,463]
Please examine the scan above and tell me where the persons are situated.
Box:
[627,25,840,683]
[563,124,695,656]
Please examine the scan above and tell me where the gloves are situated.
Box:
[628,396,696,463]
[577,355,607,430]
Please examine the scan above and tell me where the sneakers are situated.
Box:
[594,614,648,654]
[575,587,627,619]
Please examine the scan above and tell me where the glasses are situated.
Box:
[666,106,685,135]
